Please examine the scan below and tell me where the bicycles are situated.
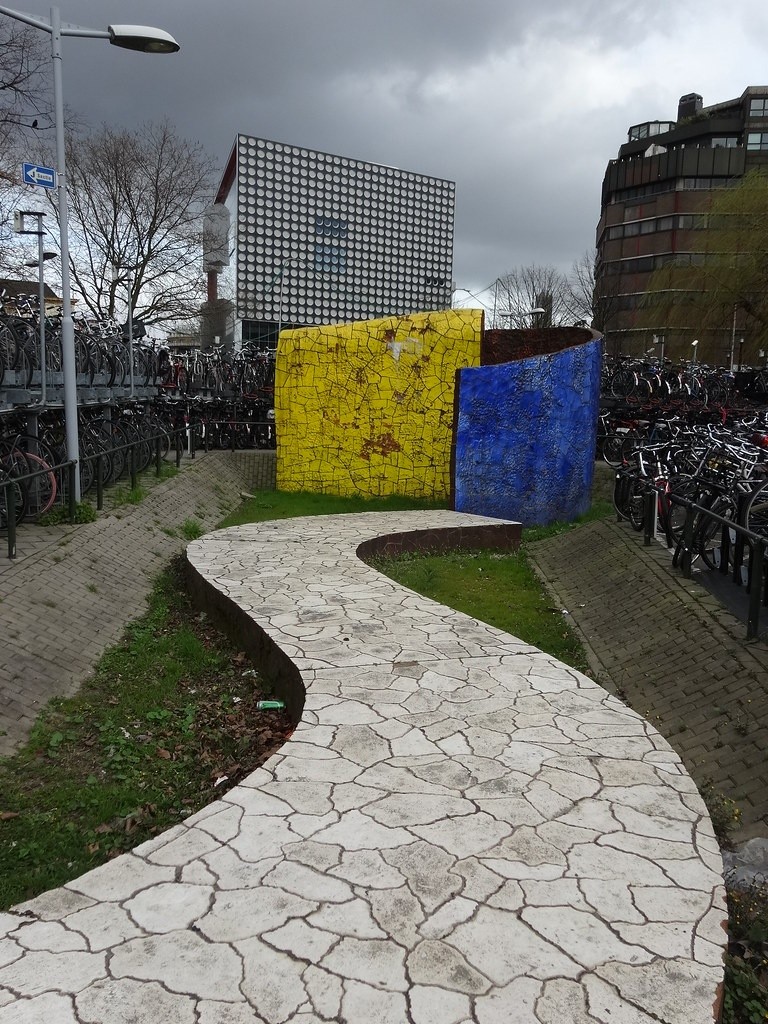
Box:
[592,349,768,603]
[0,289,277,529]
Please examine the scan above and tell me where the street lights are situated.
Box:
[498,308,545,329]
[0,4,182,512]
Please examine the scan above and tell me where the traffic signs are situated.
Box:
[21,162,56,189]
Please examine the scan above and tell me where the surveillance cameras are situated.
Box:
[691,340,699,347]
